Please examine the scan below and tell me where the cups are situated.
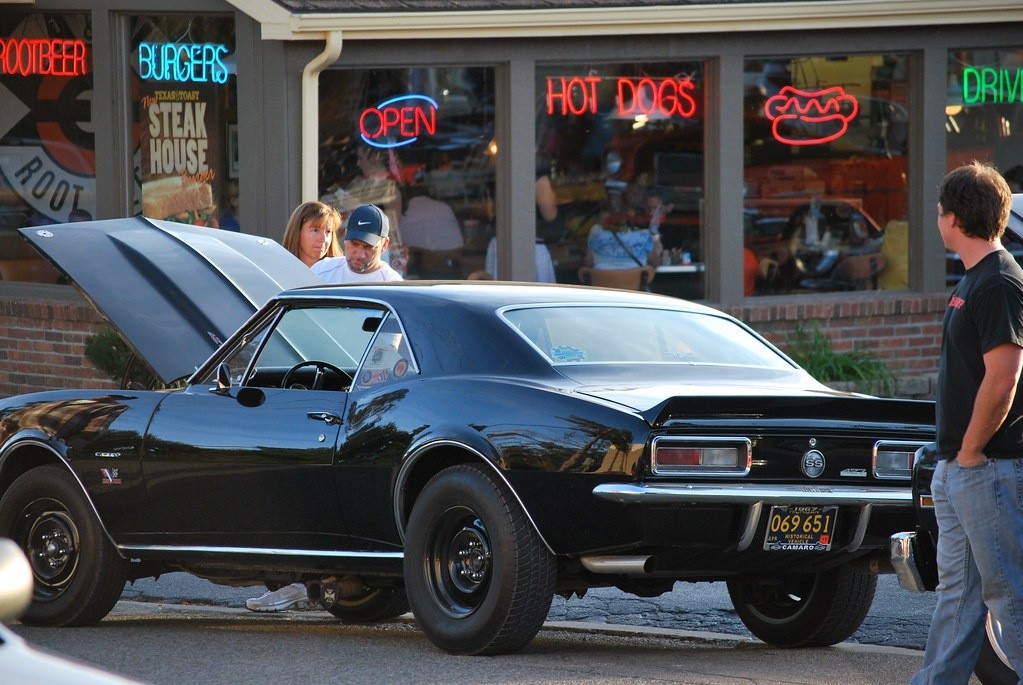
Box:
[465,220,478,237]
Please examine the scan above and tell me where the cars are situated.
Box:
[0,215,938,656]
[319,82,996,299]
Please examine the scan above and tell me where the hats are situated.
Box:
[344,203,389,246]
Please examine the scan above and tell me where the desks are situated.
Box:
[654,262,705,295]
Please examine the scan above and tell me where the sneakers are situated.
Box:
[244,582,309,612]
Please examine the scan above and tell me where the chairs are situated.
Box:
[576,264,657,294]
[800,251,888,294]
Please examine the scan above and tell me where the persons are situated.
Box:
[282,201,343,269]
[907,162,1022,685]
[219,179,240,232]
[312,120,760,298]
[309,202,405,369]
